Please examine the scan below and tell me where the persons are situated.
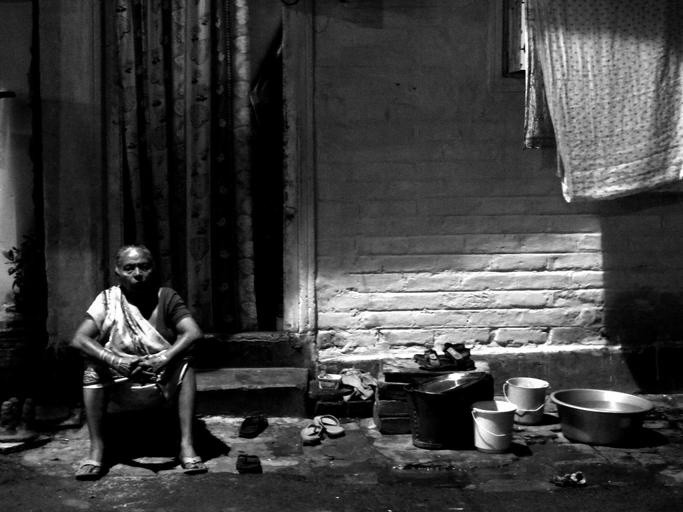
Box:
[66,242,209,480]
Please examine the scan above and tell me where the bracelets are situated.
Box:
[159,354,167,364]
[98,348,122,372]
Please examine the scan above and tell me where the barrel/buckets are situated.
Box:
[403,370,495,449]
[502,375,552,425]
[470,399,518,453]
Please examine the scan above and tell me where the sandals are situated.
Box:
[414,343,474,370]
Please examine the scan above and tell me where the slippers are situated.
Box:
[74,459,102,478]
[180,456,208,473]
[314,415,344,435]
[238,414,266,437]
[300,424,322,442]
[236,454,259,472]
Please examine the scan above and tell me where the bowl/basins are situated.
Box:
[549,388,657,446]
